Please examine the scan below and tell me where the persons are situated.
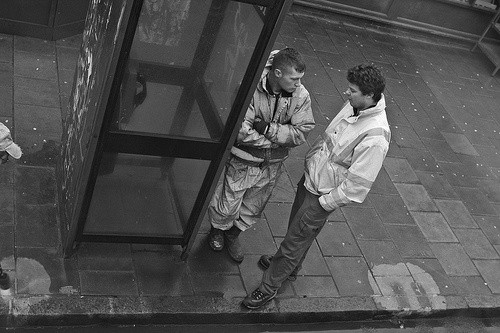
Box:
[207,48,316,261]
[242,63,391,310]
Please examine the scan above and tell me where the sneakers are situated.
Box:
[209,224,224,251]
[224,226,244,261]
[260,255,297,281]
[242,287,277,309]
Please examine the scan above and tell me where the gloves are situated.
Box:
[252,115,267,134]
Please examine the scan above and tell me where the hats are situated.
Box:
[0,122,22,159]
